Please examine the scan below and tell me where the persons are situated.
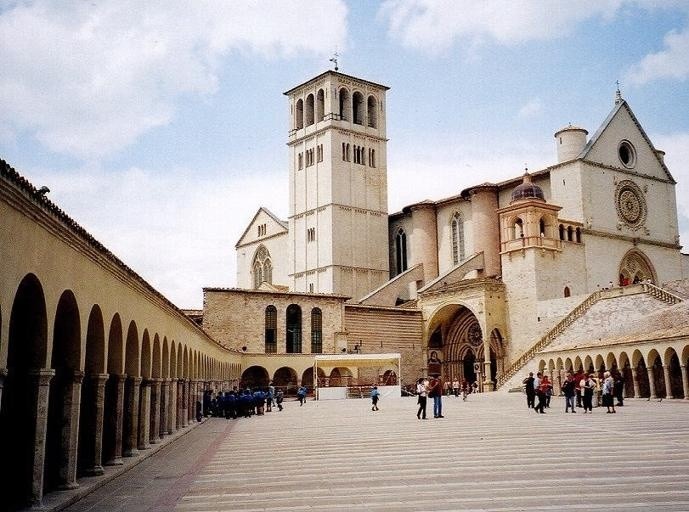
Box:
[595,282,601,293]
[196,382,308,420]
[442,377,480,402]
[523,369,625,414]
[417,377,430,420]
[428,350,445,366]
[430,374,445,418]
[608,280,615,290]
[370,385,381,411]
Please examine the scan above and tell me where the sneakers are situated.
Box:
[528,403,550,414]
[566,408,576,413]
[434,414,444,418]
[583,410,592,415]
[607,410,616,413]
[371,407,379,411]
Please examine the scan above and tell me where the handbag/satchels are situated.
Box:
[428,390,437,398]
[584,388,593,397]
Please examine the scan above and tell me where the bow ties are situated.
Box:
[417,414,427,419]
